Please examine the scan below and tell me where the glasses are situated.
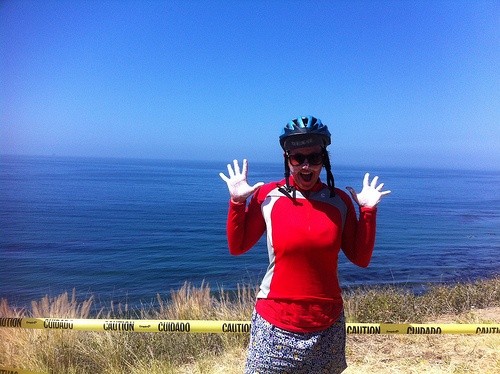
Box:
[287,153,324,166]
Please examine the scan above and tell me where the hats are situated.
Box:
[284,135,322,151]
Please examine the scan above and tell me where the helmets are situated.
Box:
[279,114,331,150]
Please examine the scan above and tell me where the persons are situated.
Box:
[219,115,392,374]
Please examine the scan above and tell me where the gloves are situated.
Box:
[219,158,264,205]
[346,172,392,208]
